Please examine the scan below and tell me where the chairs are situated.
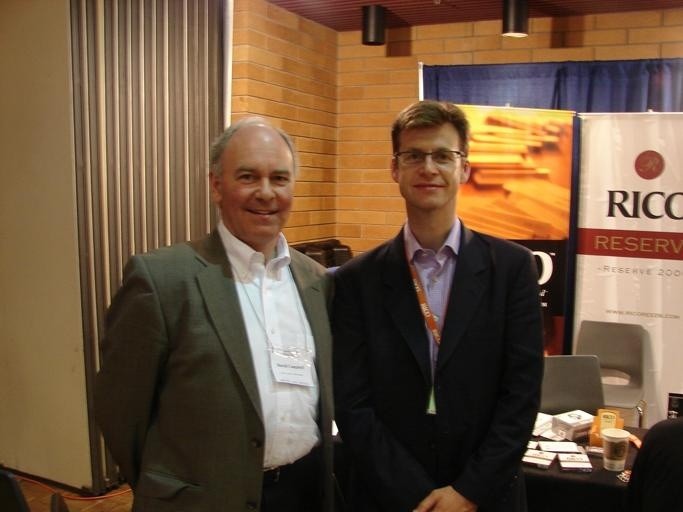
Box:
[539,354,607,416]
[574,319,648,428]
[619,418,683,511]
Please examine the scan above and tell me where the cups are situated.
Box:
[601,428,629,471]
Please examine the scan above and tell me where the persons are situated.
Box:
[329,99,544,512]
[92,115,334,511]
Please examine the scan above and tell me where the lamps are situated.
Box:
[361,5,385,45]
[501,0,529,37]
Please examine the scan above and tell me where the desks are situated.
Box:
[521,425,649,512]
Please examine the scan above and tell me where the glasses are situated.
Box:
[393,150,465,164]
